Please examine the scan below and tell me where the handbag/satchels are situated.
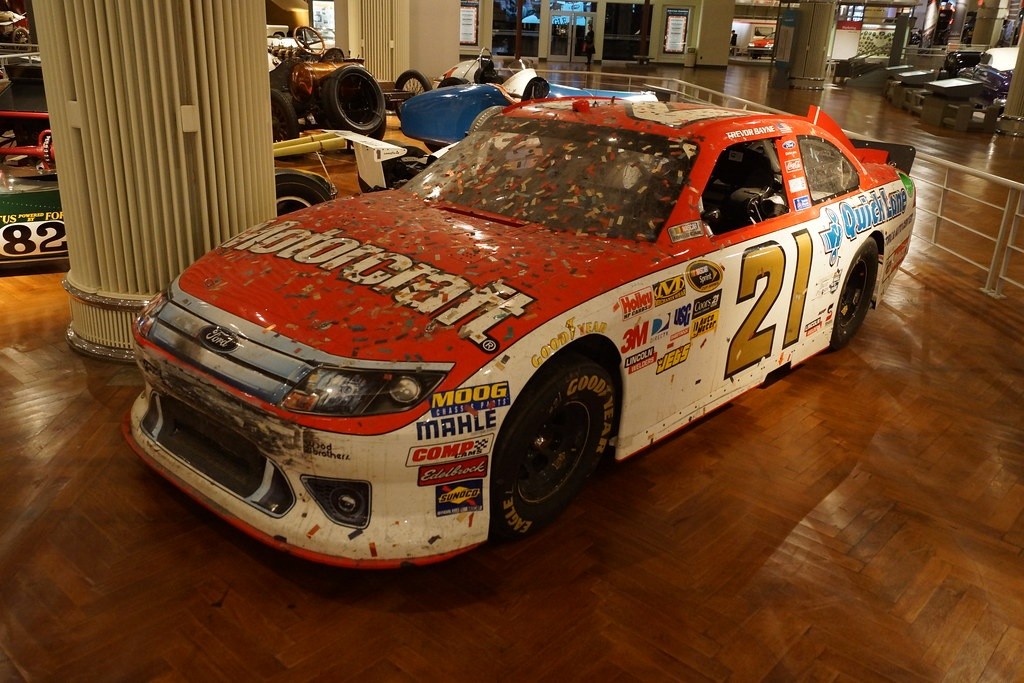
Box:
[582,43,586,52]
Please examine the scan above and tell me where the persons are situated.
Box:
[730,30,737,50]
[582,24,596,65]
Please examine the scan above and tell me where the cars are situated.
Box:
[120,96,918,570]
[748,32,776,59]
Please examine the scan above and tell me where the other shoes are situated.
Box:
[585,62,590,65]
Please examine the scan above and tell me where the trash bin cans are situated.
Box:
[684,46,697,68]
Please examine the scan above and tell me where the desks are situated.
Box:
[729,45,774,57]
